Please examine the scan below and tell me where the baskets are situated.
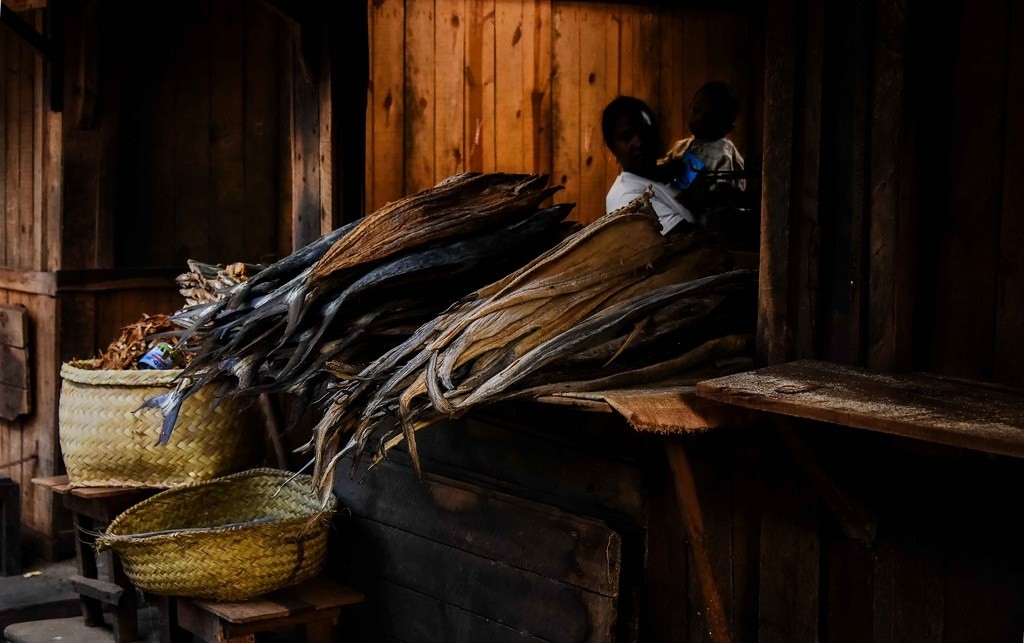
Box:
[59,359,250,489]
[104,468,330,604]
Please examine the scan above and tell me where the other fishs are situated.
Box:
[129,171,760,540]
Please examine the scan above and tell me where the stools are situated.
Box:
[166,571,367,643]
[36,475,170,643]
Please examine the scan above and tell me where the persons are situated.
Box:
[601,77,745,270]
